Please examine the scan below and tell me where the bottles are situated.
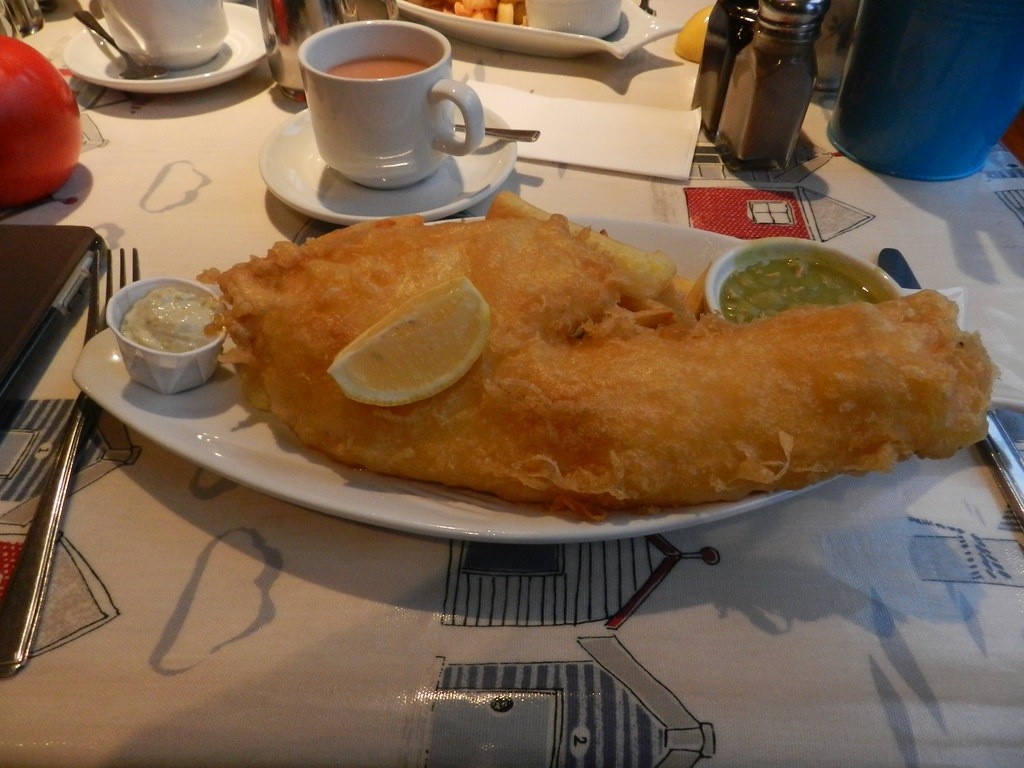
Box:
[715,0,832,170]
[691,0,758,143]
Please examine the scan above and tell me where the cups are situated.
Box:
[0,0,44,39]
[256,0,399,103]
[99,0,214,64]
[827,0,1024,182]
[298,19,483,190]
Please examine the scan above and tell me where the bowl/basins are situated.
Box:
[525,0,622,38]
[106,278,224,395]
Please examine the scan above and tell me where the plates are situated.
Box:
[396,0,684,59]
[71,218,886,537]
[62,1,275,93]
[703,237,905,323]
[258,105,517,224]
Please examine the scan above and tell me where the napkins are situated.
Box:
[454,79,702,181]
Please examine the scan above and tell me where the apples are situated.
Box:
[0,33,80,208]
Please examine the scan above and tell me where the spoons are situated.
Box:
[74,11,171,77]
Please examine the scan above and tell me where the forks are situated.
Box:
[0,247,138,677]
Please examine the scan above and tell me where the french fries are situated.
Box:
[485,190,695,317]
[428,0,528,27]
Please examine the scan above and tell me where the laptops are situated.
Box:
[0,223,106,396]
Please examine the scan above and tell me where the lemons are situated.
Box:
[326,275,491,406]
[675,4,715,63]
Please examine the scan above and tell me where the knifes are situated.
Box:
[878,248,1024,530]
